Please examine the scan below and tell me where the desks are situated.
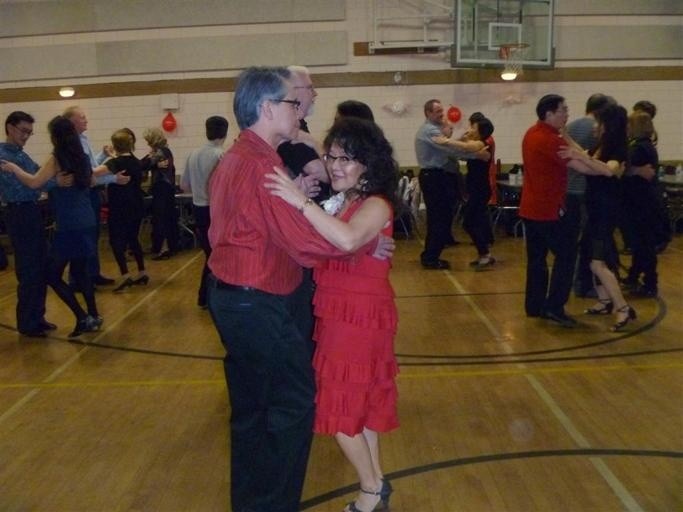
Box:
[144,192,198,249]
[657,173,683,187]
[492,179,523,240]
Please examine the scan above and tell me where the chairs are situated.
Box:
[662,187,683,234]
[392,168,425,245]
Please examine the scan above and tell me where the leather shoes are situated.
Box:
[540,308,576,325]
[471,256,495,267]
[422,259,449,269]
[17,321,56,337]
[577,288,599,298]
[69,275,115,292]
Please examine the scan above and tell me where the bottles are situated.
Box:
[658,166,664,181]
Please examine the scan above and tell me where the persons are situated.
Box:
[205,64,403,512]
[414,100,496,269]
[0,107,228,337]
[520,93,662,332]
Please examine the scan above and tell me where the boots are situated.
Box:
[619,267,641,286]
[630,273,657,296]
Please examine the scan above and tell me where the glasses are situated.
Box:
[295,85,314,91]
[322,154,356,164]
[268,99,301,110]
[14,125,33,136]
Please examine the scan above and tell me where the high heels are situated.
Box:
[151,248,170,259]
[68,315,103,337]
[584,299,613,313]
[114,275,148,290]
[343,479,392,511]
[612,304,636,332]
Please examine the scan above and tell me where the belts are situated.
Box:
[216,280,255,291]
